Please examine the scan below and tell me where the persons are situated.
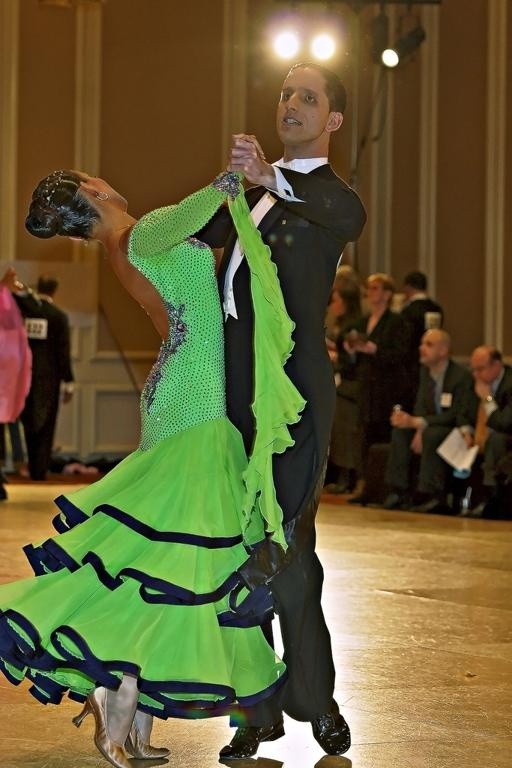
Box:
[324,265,444,503]
[382,329,511,519]
[1,135,306,765]
[20,275,75,483]
[1,265,35,500]
[188,62,365,759]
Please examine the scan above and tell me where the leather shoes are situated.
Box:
[311,709,351,755]
[219,717,285,759]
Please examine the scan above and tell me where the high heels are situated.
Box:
[71,687,132,768]
[126,715,171,760]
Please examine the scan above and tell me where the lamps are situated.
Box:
[378,3,431,70]
